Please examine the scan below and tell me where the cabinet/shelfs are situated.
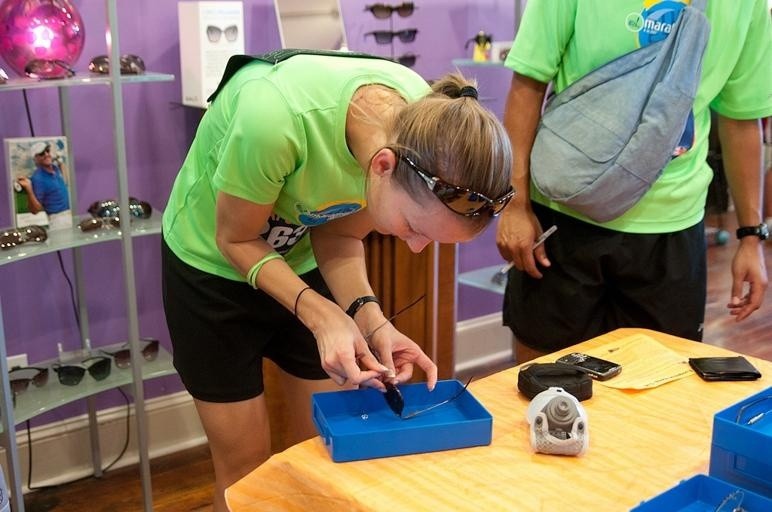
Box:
[0,1,179,511]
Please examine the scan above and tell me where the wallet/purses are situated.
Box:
[688,355,762,382]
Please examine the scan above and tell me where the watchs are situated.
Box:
[340,294,380,316]
[730,223,767,243]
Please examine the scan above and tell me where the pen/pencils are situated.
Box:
[500,226,558,275]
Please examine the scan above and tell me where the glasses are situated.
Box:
[98,202,152,225]
[367,2,416,21]
[364,27,418,46]
[4,363,50,393]
[78,214,122,233]
[361,291,477,424]
[204,24,241,44]
[388,145,517,218]
[89,195,137,215]
[89,51,146,76]
[26,59,79,83]
[48,353,113,385]
[464,27,492,50]
[98,334,165,365]
[38,149,50,156]
[0,224,48,251]
[386,53,419,70]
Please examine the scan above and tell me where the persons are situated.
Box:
[498,0,767,365]
[2,136,77,233]
[161,51,514,508]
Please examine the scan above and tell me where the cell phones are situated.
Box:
[555,351,622,381]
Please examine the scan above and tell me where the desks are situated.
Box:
[225,324,771,512]
